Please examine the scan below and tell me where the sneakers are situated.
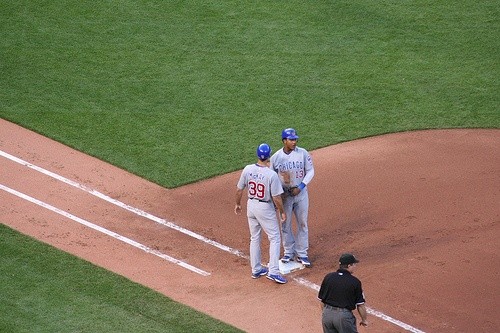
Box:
[266,274,288,283]
[252,268,268,279]
[297,256,311,265]
[281,256,292,262]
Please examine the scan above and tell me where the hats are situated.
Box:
[339,253,360,265]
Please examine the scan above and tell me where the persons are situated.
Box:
[269,128,315,265]
[234,143,288,284]
[318,253,368,333]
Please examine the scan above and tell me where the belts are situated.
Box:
[324,304,349,312]
[250,198,268,202]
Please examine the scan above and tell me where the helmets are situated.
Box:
[256,144,272,159]
[282,127,300,139]
